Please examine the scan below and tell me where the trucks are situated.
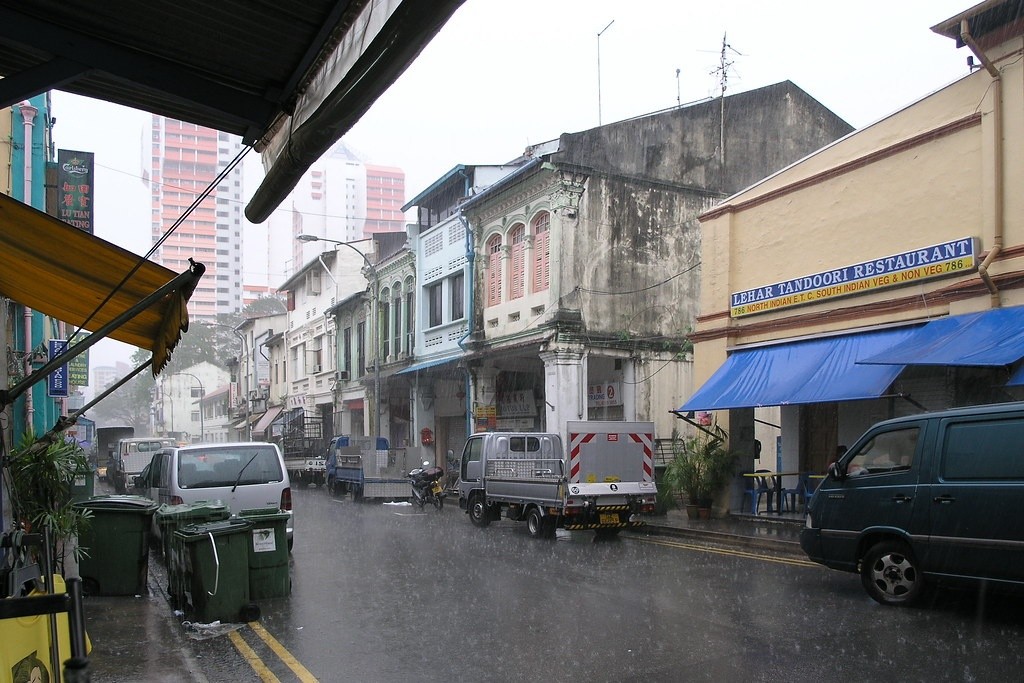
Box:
[458,421,659,539]
[96,427,134,481]
[323,434,414,503]
[277,437,327,486]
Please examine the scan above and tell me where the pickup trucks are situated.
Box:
[106,437,178,495]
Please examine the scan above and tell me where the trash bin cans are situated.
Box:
[71,493,159,597]
[172,514,262,625]
[68,464,96,505]
[154,498,231,603]
[227,506,292,600]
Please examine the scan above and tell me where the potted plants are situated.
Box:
[656,415,746,519]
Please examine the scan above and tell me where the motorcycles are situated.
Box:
[442,449,462,498]
[404,457,448,509]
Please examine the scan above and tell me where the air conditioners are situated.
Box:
[339,370,349,381]
[313,365,321,373]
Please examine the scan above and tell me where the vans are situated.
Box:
[134,441,294,561]
[799,401,1023,606]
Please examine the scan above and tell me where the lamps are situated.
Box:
[966,56,982,72]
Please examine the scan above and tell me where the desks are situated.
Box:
[808,474,827,478]
[743,472,806,514]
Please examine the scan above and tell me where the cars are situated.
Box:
[124,464,149,499]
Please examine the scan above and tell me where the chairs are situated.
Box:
[739,470,819,518]
[181,458,240,487]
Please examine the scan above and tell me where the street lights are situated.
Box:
[297,234,380,437]
[149,392,174,438]
[173,372,204,441]
[201,321,251,441]
